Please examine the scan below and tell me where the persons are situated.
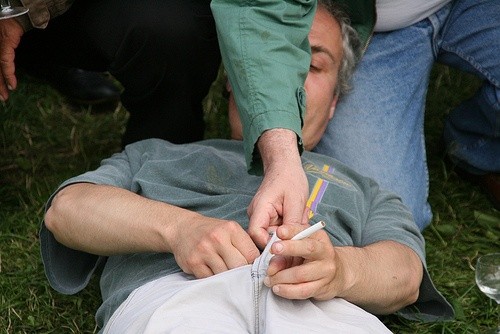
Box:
[39,0,456,333]
[0,0,220,152]
[212,0,500,234]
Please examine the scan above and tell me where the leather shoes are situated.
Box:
[39,64,121,108]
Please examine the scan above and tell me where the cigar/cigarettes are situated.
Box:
[290,221,325,239]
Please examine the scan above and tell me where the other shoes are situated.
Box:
[463,171,500,212]
